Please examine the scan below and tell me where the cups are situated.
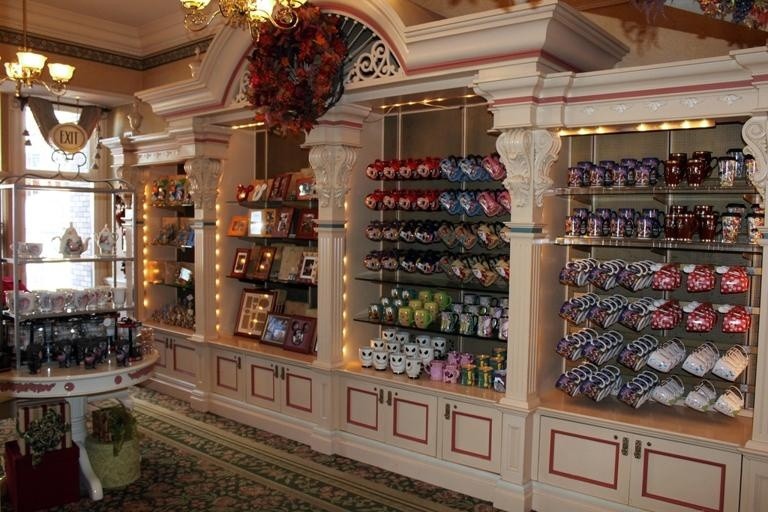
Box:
[415,334,431,346]
[475,355,490,366]
[437,222,511,248]
[494,369,506,392]
[567,159,666,187]
[439,258,509,287]
[406,358,423,379]
[745,155,756,187]
[424,302,444,320]
[499,297,508,307]
[383,305,397,324]
[364,190,437,211]
[381,328,397,343]
[398,307,415,327]
[500,307,508,319]
[650,298,751,337]
[390,287,402,298]
[419,291,432,301]
[370,338,386,352]
[746,204,763,245]
[459,312,479,337]
[477,366,494,388]
[493,348,507,360]
[403,343,419,358]
[486,307,504,319]
[418,346,441,366]
[440,155,504,181]
[402,289,413,307]
[440,309,459,333]
[358,347,373,369]
[720,213,742,244]
[489,357,505,369]
[476,315,498,337]
[565,207,663,239]
[365,220,436,245]
[718,156,736,188]
[373,352,390,371]
[647,337,748,382]
[498,316,508,342]
[379,296,391,305]
[724,149,745,180]
[444,364,460,384]
[4,286,127,312]
[389,352,406,375]
[364,249,446,274]
[463,294,478,304]
[423,359,443,380]
[450,302,467,320]
[650,261,748,296]
[362,157,441,180]
[664,205,721,243]
[557,255,653,290]
[10,242,28,257]
[465,304,487,315]
[479,295,499,307]
[368,303,383,321]
[408,300,424,310]
[395,331,415,352]
[26,243,44,257]
[392,298,405,307]
[445,350,461,367]
[430,336,448,359]
[459,353,474,364]
[414,311,432,329]
[438,190,512,216]
[554,363,656,408]
[651,375,745,417]
[667,148,718,188]
[558,293,656,333]
[433,291,452,310]
[385,342,401,361]
[554,326,657,373]
[461,364,477,386]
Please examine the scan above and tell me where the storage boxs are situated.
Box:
[15,398,73,457]
[4,438,80,512]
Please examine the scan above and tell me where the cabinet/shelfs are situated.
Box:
[339,159,509,504]
[143,196,195,408]
[536,136,767,512]
[208,194,318,446]
[0,154,160,503]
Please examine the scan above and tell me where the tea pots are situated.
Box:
[94,224,119,257]
[52,220,93,259]
[9,318,144,365]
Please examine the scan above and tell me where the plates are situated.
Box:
[16,257,47,260]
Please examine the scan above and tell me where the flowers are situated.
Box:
[244,5,349,138]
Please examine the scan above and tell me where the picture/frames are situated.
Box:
[228,172,319,357]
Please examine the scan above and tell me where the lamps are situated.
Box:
[179,0,307,41]
[0,0,76,100]
